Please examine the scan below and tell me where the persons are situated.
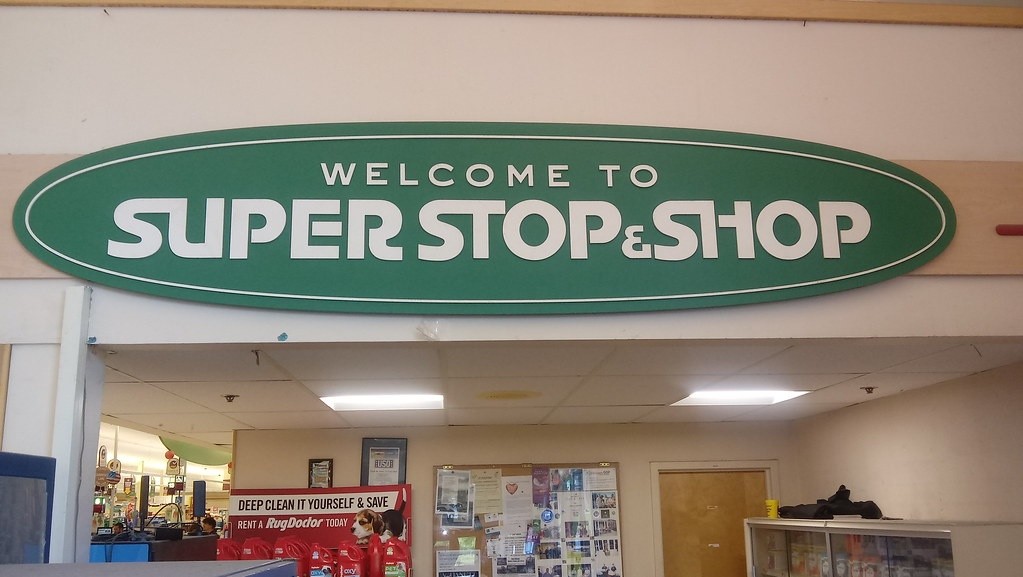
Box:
[577,524,589,537]
[202,518,220,539]
[597,494,615,508]
[545,547,557,559]
[552,470,560,489]
[543,568,551,577]
[114,523,123,533]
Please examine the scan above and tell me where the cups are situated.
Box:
[765,500,779,518]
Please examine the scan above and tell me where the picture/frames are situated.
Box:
[359,437,407,486]
[308,458,333,488]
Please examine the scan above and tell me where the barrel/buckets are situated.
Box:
[217,535,412,577]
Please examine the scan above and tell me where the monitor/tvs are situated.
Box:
[96,527,111,536]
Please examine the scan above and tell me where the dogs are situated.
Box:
[350,488,407,545]
